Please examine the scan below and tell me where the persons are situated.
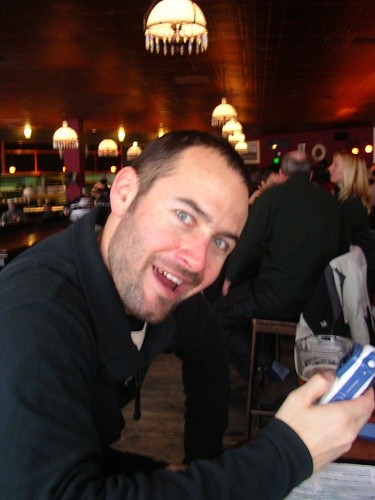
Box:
[0,149,375,472]
[0,129,374,500]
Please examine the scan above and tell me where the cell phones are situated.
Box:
[317,341,375,404]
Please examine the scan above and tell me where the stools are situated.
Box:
[241,318,296,445]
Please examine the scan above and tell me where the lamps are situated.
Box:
[97,139,118,159]
[52,121,79,152]
[210,98,248,151]
[145,0,209,58]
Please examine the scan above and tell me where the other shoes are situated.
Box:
[252,363,273,403]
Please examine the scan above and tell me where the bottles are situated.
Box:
[0,249,8,267]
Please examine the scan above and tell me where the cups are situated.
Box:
[294,335,354,406]
